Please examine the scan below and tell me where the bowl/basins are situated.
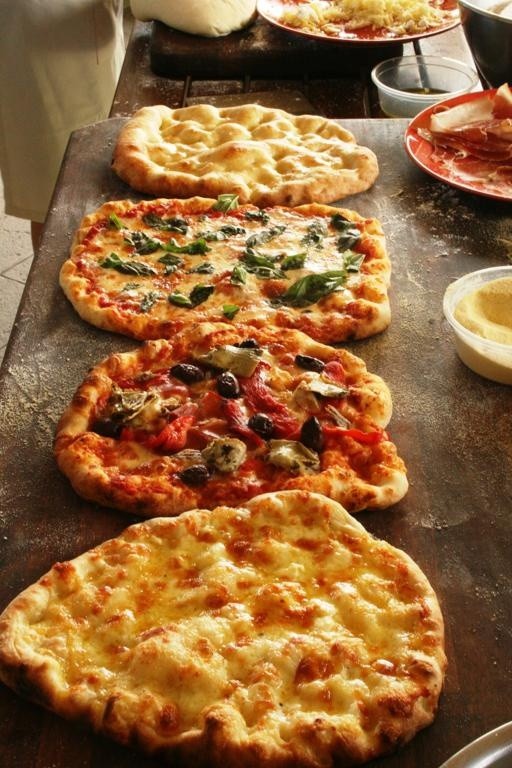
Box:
[370,54,480,119]
[442,266,512,384]
[457,0,512,89]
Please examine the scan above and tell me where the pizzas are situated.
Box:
[56,195,393,341]
[108,102,380,208]
[1,489,449,764]
[51,319,411,517]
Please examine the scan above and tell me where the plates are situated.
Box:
[254,0,468,47]
[431,720,512,766]
[403,88,512,202]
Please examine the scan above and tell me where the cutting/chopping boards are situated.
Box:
[150,13,404,81]
[0,109,512,766]
